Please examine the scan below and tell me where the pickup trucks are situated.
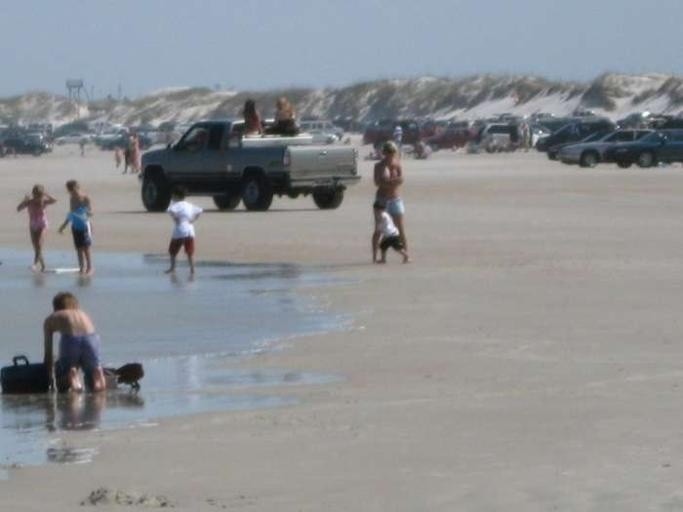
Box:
[138,118,360,210]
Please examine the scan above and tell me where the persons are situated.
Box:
[13,180,57,275]
[74,273,89,289]
[264,95,301,135]
[371,199,409,264]
[166,273,196,287]
[45,391,105,464]
[52,176,95,276]
[30,270,47,287]
[370,138,408,262]
[40,290,107,393]
[161,182,204,275]
[364,108,657,162]
[0,124,143,174]
[227,99,263,140]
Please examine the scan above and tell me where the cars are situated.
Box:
[0,122,165,157]
